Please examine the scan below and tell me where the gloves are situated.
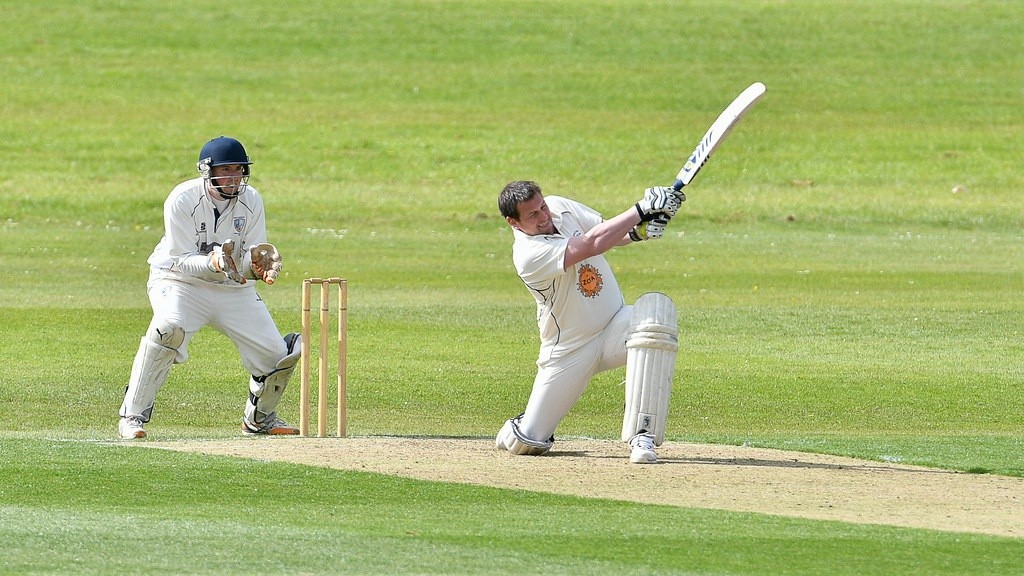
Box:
[249,243,283,285]
[644,186,686,215]
[213,239,246,285]
[634,214,671,242]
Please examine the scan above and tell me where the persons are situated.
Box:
[118,137,301,438]
[495,180,687,465]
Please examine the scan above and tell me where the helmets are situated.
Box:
[196,137,253,197]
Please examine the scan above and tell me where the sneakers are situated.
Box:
[119,417,147,438]
[628,433,657,463]
[242,422,300,436]
[495,418,518,448]
